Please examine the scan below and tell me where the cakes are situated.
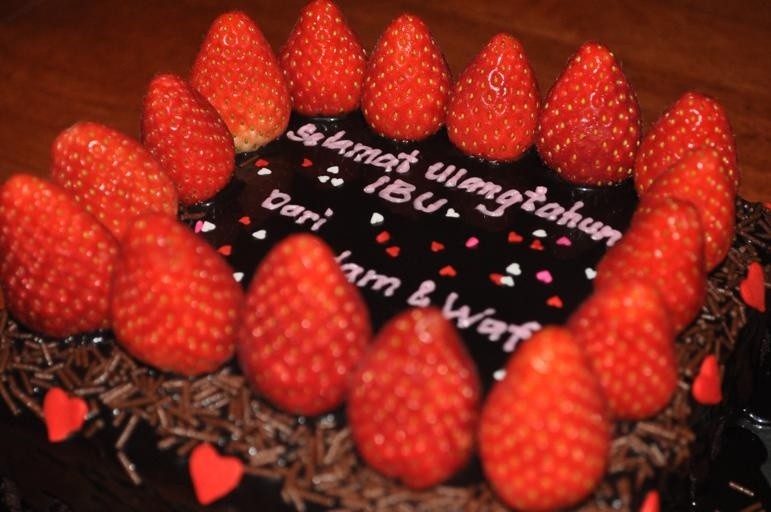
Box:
[0,0,739,512]
